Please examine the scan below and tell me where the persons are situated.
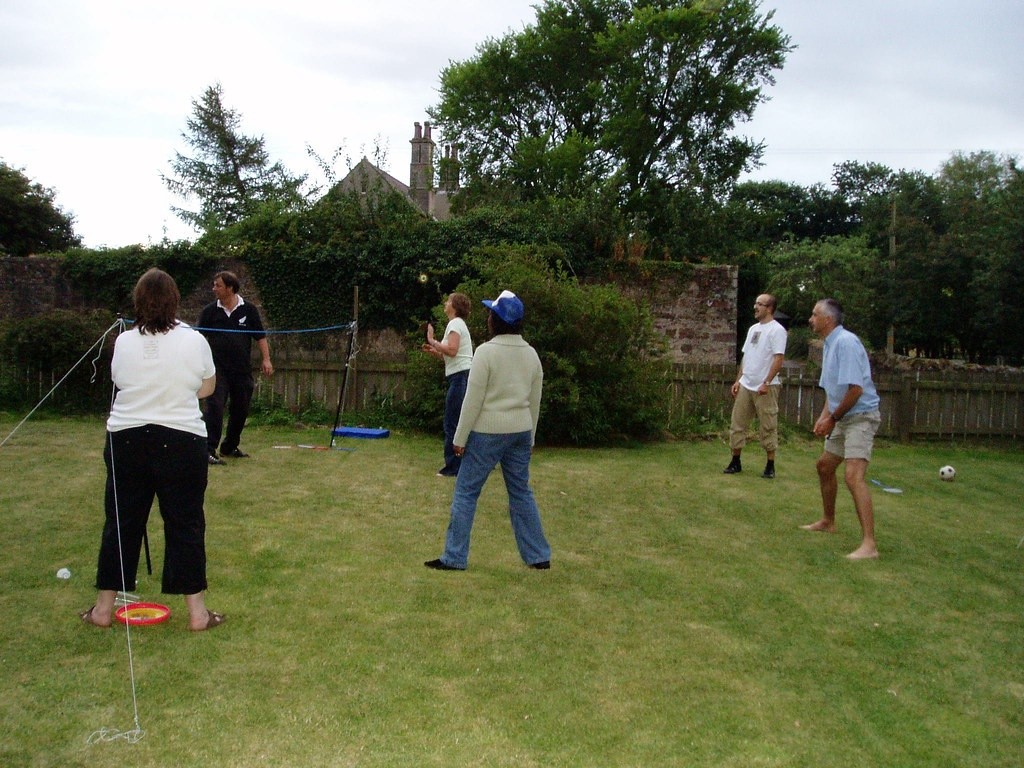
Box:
[198,272,272,465]
[724,294,787,478]
[798,299,881,558]
[422,294,473,476]
[81,270,228,631]
[424,291,552,570]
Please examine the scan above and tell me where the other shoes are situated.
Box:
[219,447,248,458]
[425,559,466,572]
[437,471,457,477]
[724,460,742,473]
[535,561,550,569]
[762,467,775,478]
[208,453,225,465]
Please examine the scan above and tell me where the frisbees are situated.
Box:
[115,603,170,626]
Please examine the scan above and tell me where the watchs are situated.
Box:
[764,381,771,386]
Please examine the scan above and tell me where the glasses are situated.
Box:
[754,301,772,308]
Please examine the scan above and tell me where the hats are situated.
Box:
[482,289,524,326]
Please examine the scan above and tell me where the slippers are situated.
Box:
[191,609,225,632]
[81,606,114,628]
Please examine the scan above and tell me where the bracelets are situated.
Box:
[832,414,839,422]
[433,340,437,347]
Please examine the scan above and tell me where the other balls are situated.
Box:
[57,568,71,580]
